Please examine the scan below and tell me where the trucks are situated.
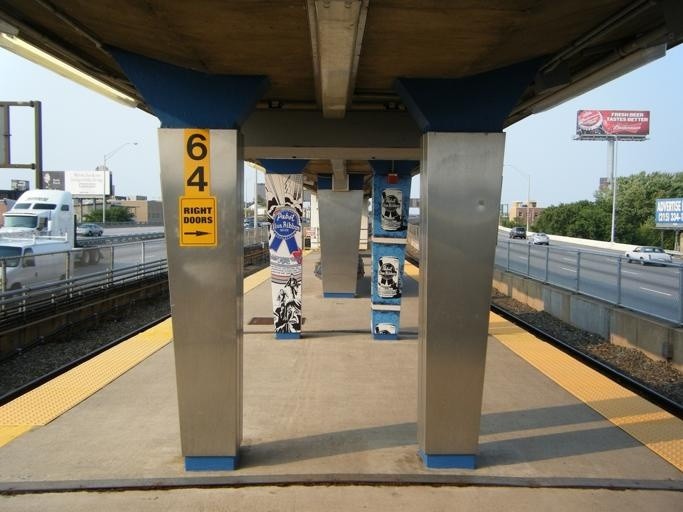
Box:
[0,188,103,268]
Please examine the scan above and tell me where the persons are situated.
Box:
[273,303,285,322]
[277,289,289,302]
[285,273,299,299]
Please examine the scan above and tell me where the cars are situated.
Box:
[243,216,261,229]
[623,245,673,268]
[74,223,106,237]
[527,232,550,247]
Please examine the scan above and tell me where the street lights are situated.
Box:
[99,139,140,222]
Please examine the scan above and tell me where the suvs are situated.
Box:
[509,226,526,240]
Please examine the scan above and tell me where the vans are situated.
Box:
[0,233,74,310]
